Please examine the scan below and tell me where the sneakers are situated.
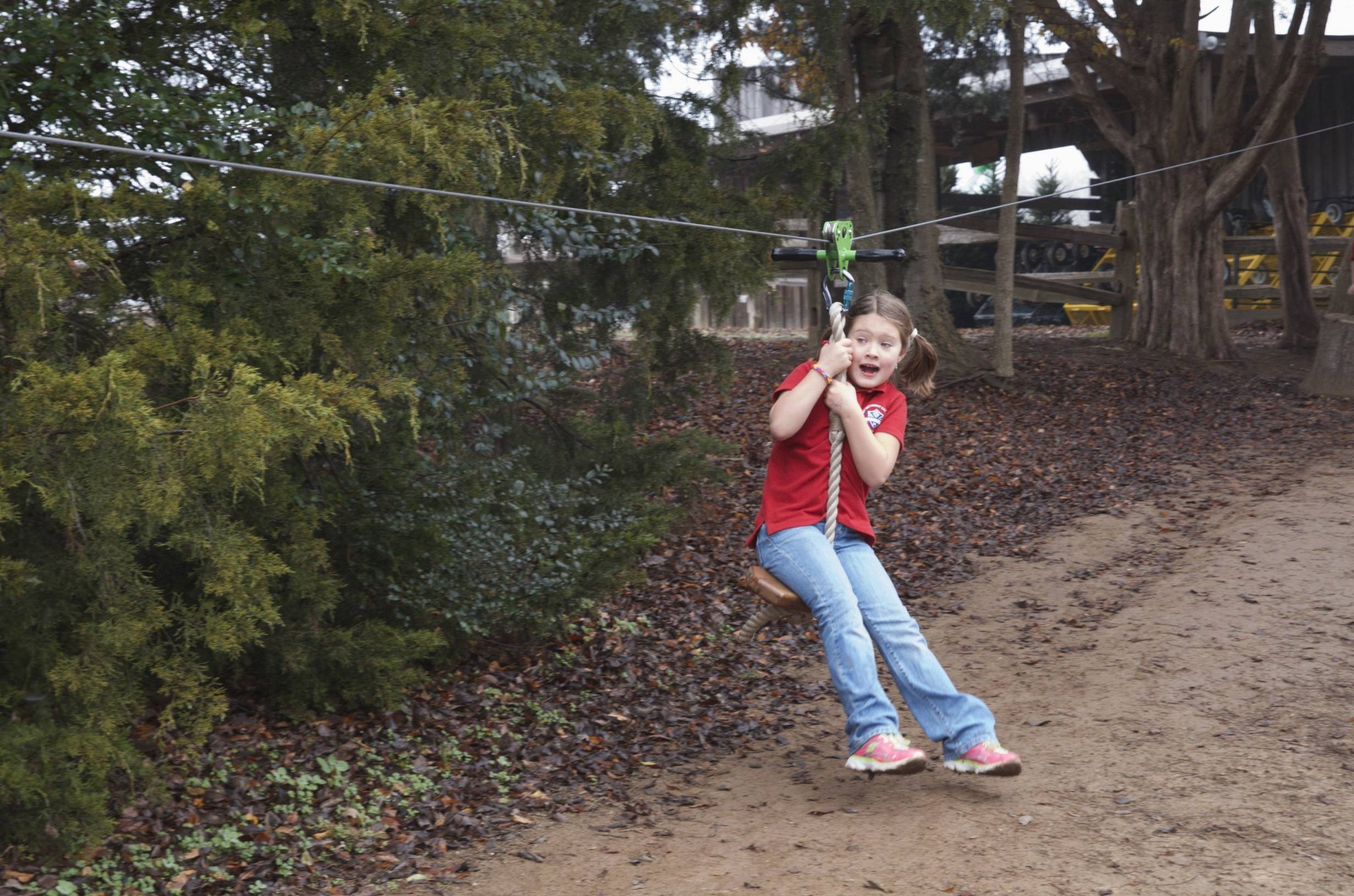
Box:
[943,739,1022,778]
[844,732,926,775]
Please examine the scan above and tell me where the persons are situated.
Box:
[745,289,1022,778]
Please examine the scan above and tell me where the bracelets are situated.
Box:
[810,364,832,383]
